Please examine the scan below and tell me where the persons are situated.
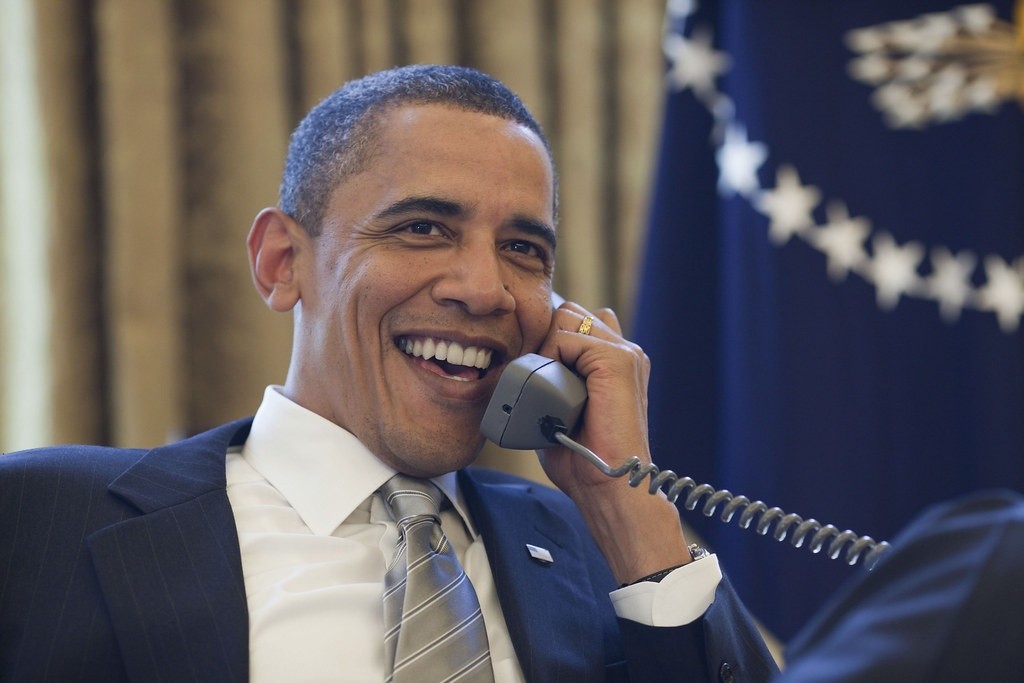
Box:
[1,64,1024,683]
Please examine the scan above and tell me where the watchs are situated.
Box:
[618,544,711,589]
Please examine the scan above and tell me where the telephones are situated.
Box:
[482,285,608,455]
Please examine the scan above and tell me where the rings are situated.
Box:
[577,315,593,335]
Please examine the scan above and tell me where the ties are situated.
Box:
[378,472,495,683]
